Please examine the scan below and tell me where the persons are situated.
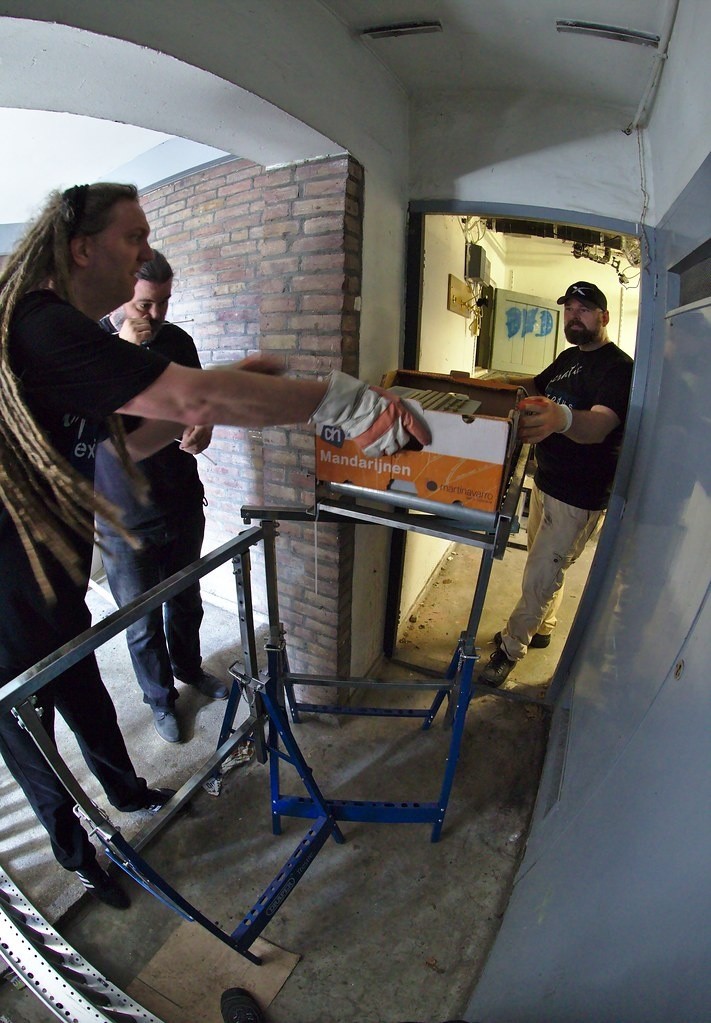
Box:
[95,248,230,742]
[0,183,431,910]
[583,310,710,675]
[479,281,635,687]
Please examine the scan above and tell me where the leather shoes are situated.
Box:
[153,709,184,744]
[189,670,230,700]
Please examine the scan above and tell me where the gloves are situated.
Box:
[307,370,431,459]
[519,395,573,444]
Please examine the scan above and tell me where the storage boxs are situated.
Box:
[315,368,523,512]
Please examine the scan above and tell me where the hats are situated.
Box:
[557,281,607,312]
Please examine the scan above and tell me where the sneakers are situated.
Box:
[220,988,264,1023]
[75,859,131,911]
[494,631,551,648]
[145,787,194,817]
[478,649,517,688]
